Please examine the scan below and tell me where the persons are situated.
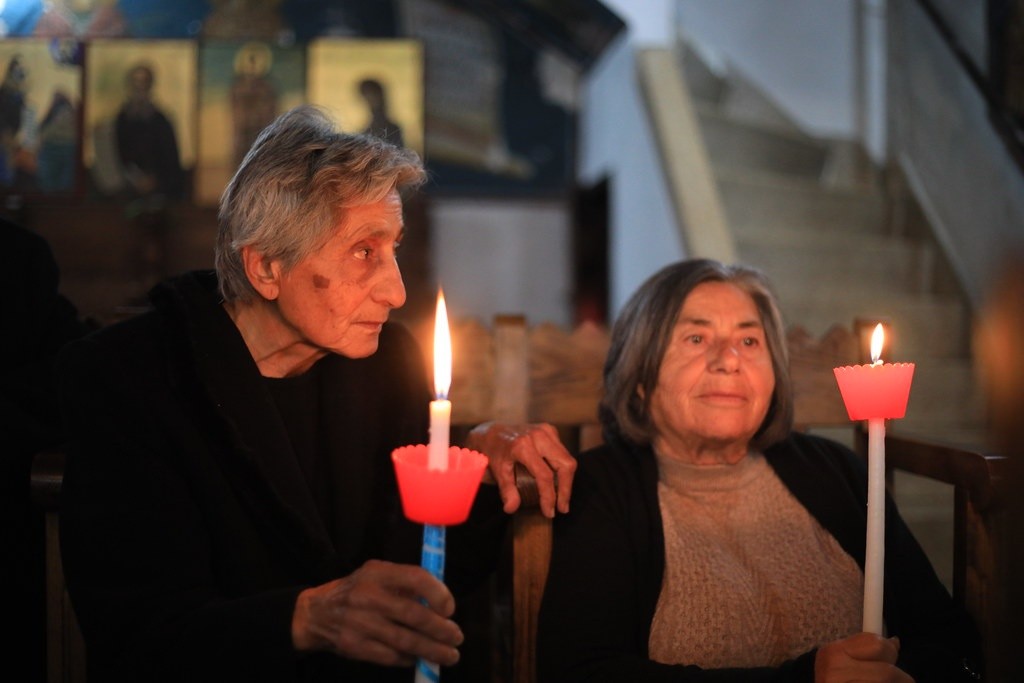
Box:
[58,106,577,682]
[534,258,956,683]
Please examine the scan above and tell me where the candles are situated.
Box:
[416,288,453,681]
[862,323,886,638]
